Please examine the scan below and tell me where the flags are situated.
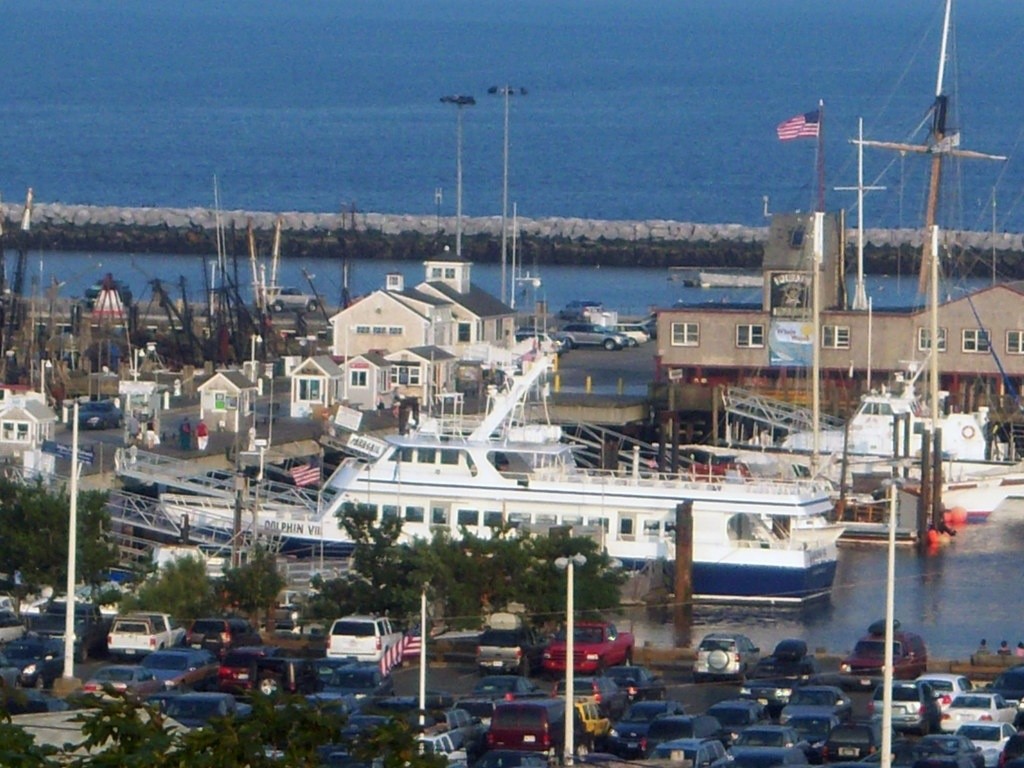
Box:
[777,108,822,140]
[516,348,536,370]
[290,458,320,489]
[379,621,421,680]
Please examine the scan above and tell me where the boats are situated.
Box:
[158,351,1024,609]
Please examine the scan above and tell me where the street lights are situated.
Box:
[486,82,530,306]
[438,94,478,261]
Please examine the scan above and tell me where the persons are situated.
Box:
[926,691,947,734]
[376,387,401,419]
[977,638,1024,656]
[127,415,209,450]
[1002,695,1024,768]
[290,608,312,634]
[761,426,769,445]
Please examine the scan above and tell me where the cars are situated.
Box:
[634,318,659,338]
[0,585,1024,768]
[66,400,122,431]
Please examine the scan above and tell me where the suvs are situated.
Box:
[600,323,650,347]
[514,330,565,357]
[261,286,319,313]
[562,299,608,323]
[558,322,628,351]
